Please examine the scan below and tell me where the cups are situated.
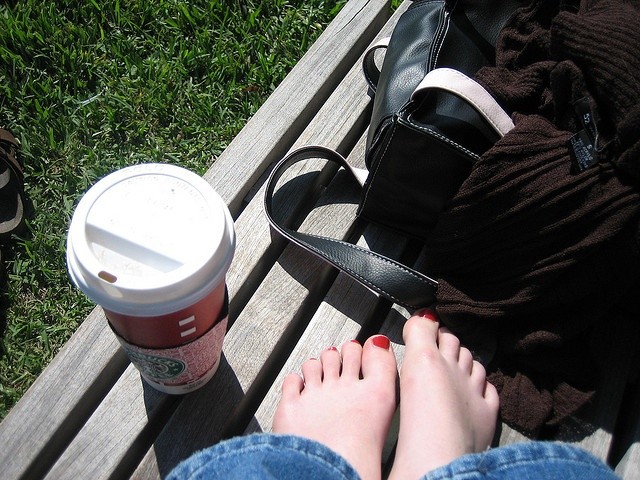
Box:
[66,162,236,395]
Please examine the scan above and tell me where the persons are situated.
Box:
[161,309,616,479]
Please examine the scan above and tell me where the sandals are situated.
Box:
[0,129,26,234]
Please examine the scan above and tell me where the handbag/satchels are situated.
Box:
[266,1,536,308]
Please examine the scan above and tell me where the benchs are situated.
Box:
[1,1,640,480]
